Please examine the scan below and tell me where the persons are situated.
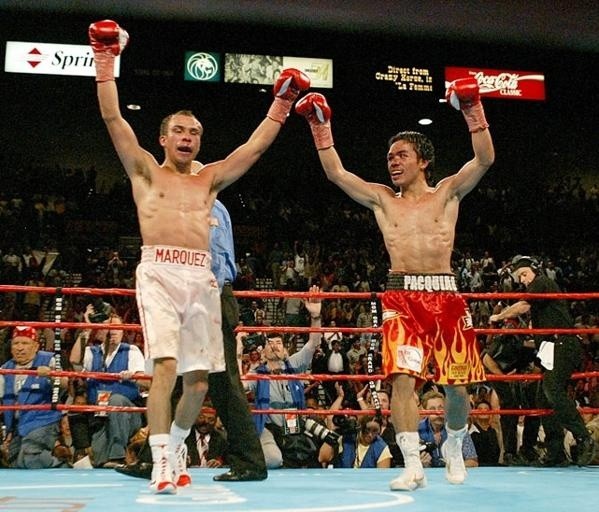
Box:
[1,160,598,483]
[295,77,498,492]
[88,17,312,495]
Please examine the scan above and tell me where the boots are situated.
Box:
[148,434,176,494]
[441,422,468,484]
[169,422,191,486]
[390,432,427,491]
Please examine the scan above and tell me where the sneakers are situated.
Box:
[575,434,595,466]
[503,446,568,468]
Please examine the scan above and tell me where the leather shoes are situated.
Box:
[214,468,268,481]
[115,460,152,479]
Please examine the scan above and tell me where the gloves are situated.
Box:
[265,68,310,126]
[88,20,130,83]
[295,92,335,150]
[445,77,489,133]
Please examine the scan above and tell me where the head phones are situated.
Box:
[519,258,538,271]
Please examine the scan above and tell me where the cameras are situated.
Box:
[304,415,340,447]
[242,334,266,347]
[332,413,358,431]
[85,287,112,323]
